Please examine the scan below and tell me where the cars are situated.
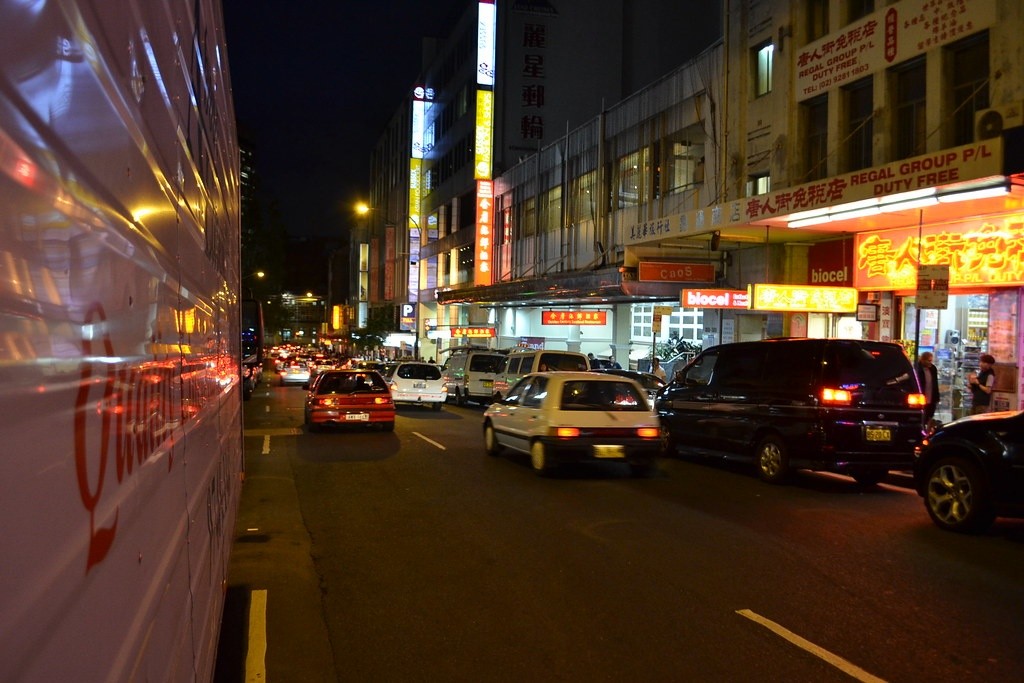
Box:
[587,369,666,411]
[383,360,448,411]
[271,342,400,386]
[483,370,662,476]
[302,368,396,431]
[280,362,311,385]
[911,409,1024,534]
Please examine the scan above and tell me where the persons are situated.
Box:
[967,354,996,415]
[605,356,622,369]
[422,357,426,363]
[428,356,436,363]
[538,362,549,372]
[588,353,600,369]
[916,352,940,425]
[648,358,667,382]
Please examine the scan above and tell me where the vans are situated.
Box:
[652,338,925,485]
[491,349,592,405]
[438,347,512,407]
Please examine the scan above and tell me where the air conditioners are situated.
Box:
[974,99,1024,142]
